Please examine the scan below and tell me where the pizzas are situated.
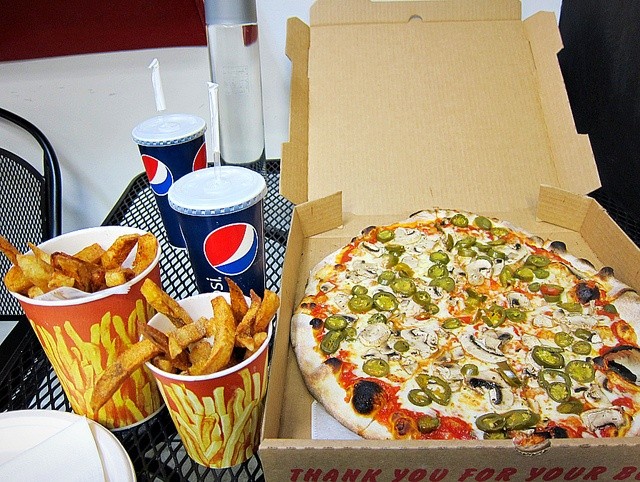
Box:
[288,206,640,455]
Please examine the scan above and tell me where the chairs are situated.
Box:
[0,108,60,346]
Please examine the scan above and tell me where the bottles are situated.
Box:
[203,0,268,188]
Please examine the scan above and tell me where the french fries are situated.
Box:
[87,276,282,413]
[0,232,166,298]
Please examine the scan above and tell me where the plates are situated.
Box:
[1,409,137,481]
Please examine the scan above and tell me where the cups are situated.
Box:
[140,291,273,470]
[168,166,267,301]
[7,226,165,432]
[131,113,207,251]
